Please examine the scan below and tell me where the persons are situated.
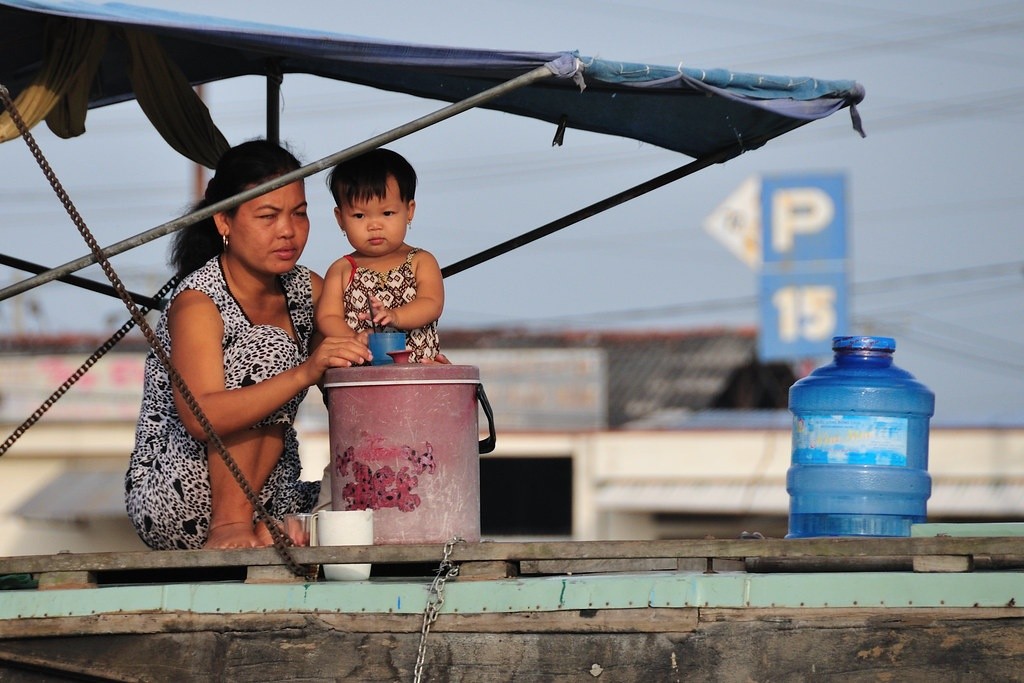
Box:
[315,148,444,364]
[122,135,451,552]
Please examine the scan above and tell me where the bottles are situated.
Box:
[785,335,934,539]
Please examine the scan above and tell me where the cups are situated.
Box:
[280,513,321,580]
[367,333,406,365]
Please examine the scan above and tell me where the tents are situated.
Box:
[0,0,866,515]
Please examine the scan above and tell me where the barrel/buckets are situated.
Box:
[324,362,496,547]
